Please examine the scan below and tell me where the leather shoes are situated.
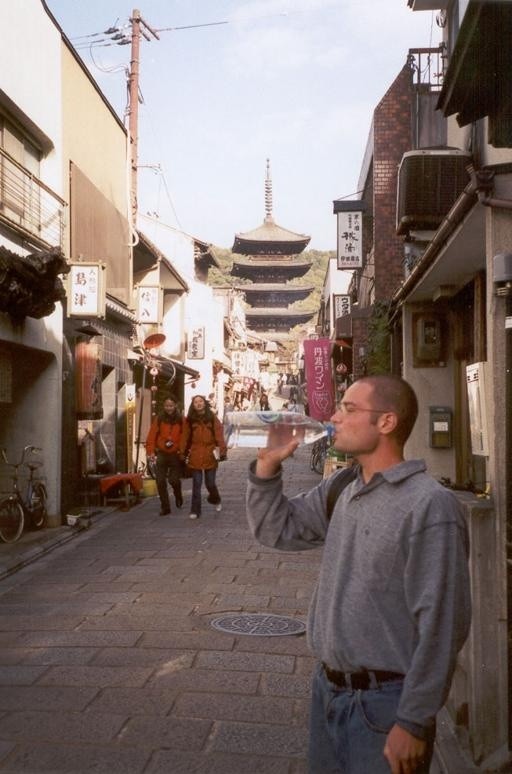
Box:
[160,508,171,516]
[176,496,183,508]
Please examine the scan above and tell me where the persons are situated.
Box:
[242,371,474,774]
[146,394,187,517]
[208,366,306,425]
[178,393,228,520]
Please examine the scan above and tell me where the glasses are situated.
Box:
[334,402,389,416]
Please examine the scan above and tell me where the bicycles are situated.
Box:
[0,444,51,545]
[308,434,331,477]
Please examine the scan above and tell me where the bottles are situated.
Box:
[220,410,337,449]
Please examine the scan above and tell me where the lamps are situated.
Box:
[491,249,511,299]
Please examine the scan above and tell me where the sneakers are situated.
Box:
[189,513,198,520]
[215,504,222,512]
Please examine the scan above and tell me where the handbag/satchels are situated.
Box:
[213,447,221,461]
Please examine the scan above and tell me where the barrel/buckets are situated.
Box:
[143,478,157,496]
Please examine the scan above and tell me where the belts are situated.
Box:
[321,662,403,690]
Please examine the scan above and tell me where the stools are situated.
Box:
[103,473,140,510]
